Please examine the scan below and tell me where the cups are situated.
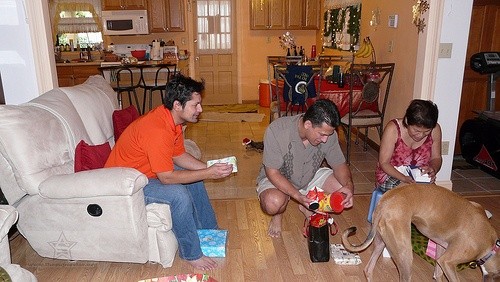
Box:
[153,42,162,60]
[338,72,344,88]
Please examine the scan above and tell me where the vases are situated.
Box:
[286,47,290,57]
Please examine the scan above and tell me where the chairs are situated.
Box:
[267,54,395,166]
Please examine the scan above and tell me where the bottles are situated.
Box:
[60,43,95,52]
[286,48,291,65]
[299,46,303,56]
[293,46,297,56]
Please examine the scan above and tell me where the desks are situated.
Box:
[270,78,379,143]
[272,60,350,70]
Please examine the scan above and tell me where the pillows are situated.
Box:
[112,104,140,143]
[74,140,111,173]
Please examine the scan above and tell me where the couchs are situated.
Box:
[0,74,202,267]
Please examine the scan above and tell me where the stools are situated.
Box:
[98,63,177,114]
[367,188,385,222]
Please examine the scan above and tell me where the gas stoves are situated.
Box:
[101,43,152,68]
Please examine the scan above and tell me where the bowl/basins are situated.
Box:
[130,50,145,60]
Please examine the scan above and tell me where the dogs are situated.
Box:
[341,184,500,282]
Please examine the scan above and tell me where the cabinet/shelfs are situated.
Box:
[248,0,321,30]
[101,0,147,10]
[146,0,186,33]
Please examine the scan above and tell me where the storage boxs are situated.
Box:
[331,243,363,266]
[197,229,227,258]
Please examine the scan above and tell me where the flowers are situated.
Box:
[278,31,296,50]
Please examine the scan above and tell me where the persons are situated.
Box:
[256,98,356,239]
[375,99,443,193]
[103,74,233,272]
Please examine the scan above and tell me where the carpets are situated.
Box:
[199,104,266,122]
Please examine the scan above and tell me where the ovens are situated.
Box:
[101,9,149,36]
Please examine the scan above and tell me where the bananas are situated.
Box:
[353,38,372,58]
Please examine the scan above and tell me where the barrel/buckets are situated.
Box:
[317,192,344,214]
